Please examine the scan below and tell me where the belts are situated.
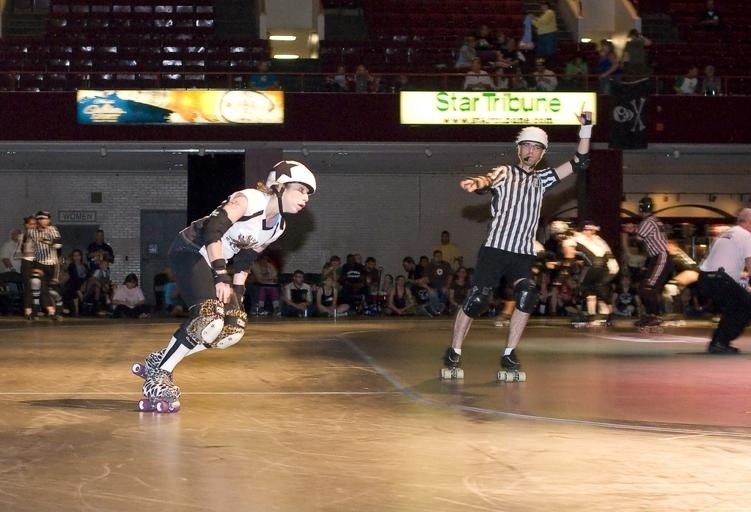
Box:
[698,273,729,279]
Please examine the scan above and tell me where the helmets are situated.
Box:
[514,124,548,150]
[638,196,655,215]
[35,211,51,220]
[549,219,571,237]
[266,158,317,197]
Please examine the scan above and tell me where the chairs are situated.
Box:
[320,0,601,93]
[618,1,747,91]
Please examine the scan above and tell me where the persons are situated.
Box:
[673,67,698,97]
[697,206,751,354]
[130,160,316,414]
[155,264,191,318]
[1,209,113,321]
[701,65,721,93]
[533,219,646,327]
[247,60,279,90]
[622,197,670,334]
[455,2,652,91]
[111,273,150,318]
[443,111,592,368]
[243,249,517,326]
[323,64,413,93]
[660,240,700,321]
[697,1,719,30]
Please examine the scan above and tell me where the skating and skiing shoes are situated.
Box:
[496,351,528,384]
[595,312,613,327]
[572,313,600,328]
[634,314,664,335]
[24,309,35,320]
[131,348,166,381]
[137,370,182,412]
[440,346,465,381]
[47,311,63,322]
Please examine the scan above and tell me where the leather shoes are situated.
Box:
[709,340,740,354]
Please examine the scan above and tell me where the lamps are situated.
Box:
[257,0,319,43]
[561,1,642,47]
[268,43,320,59]
